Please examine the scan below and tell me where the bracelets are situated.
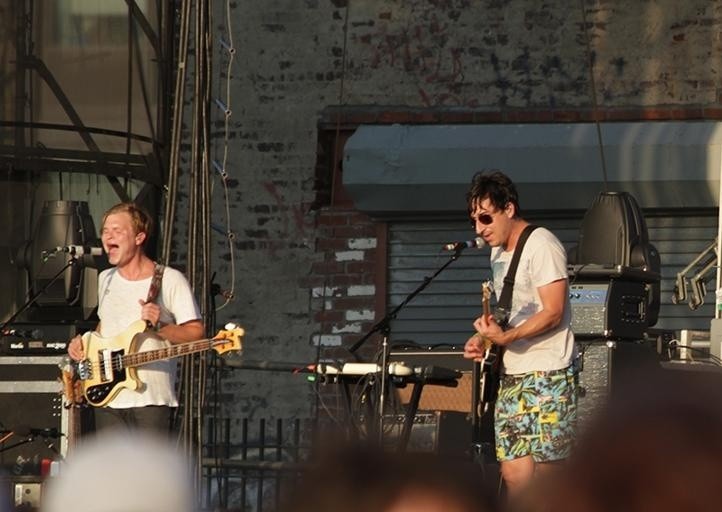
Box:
[474,332,486,344]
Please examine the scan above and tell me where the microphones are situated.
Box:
[11,423,63,441]
[445,237,484,253]
[58,245,103,258]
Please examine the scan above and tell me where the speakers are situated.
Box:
[585,189,664,332]
[32,199,99,306]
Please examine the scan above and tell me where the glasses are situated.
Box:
[472,213,493,227]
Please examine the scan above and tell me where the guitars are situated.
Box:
[479,279,497,402]
[58,323,247,408]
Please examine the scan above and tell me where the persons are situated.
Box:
[68,197,206,440]
[541,362,722,512]
[459,169,576,512]
[35,424,194,511]
[284,443,471,512]
